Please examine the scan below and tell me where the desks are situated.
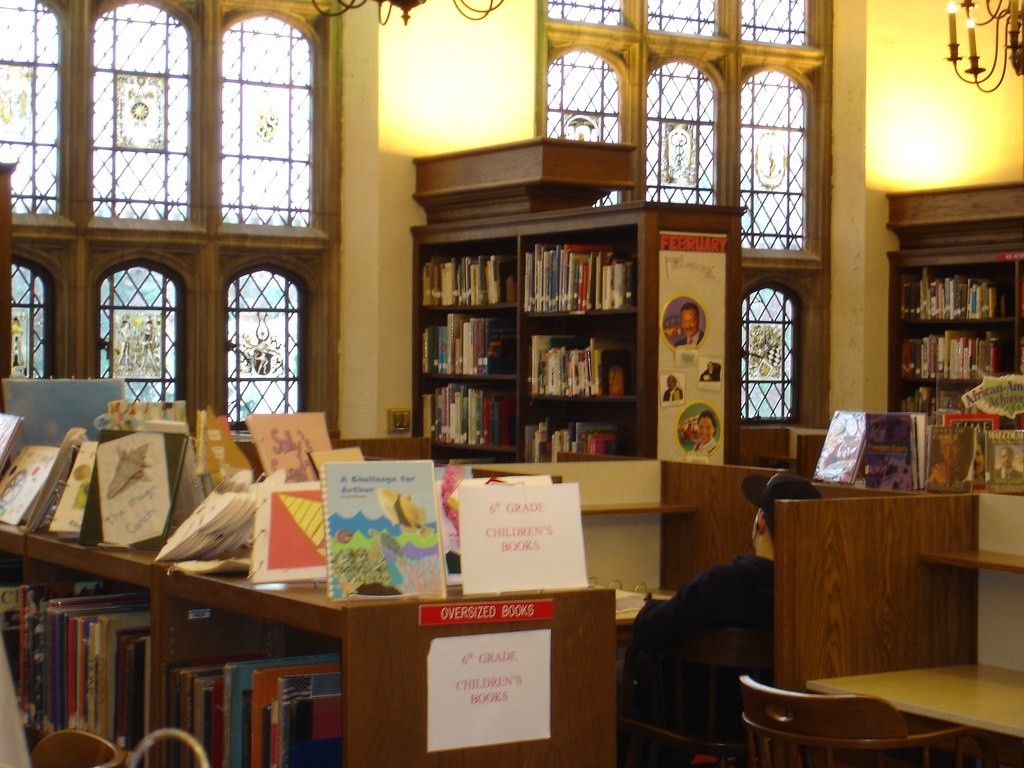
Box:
[615,587,679,627]
[807,664,1024,742]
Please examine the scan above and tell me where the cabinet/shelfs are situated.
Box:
[0,523,619,768]
[886,240,1024,430]
[410,200,742,464]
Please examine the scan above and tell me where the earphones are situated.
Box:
[755,523,760,531]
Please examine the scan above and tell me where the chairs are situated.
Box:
[619,623,773,768]
[740,674,1001,768]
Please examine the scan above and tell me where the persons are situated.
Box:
[928,432,984,488]
[700,362,720,381]
[691,411,718,457]
[673,303,705,348]
[626,472,822,768]
[994,448,1023,485]
[663,374,683,401]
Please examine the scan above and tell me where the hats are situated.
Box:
[742,472,822,522]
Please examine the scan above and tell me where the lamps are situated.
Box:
[947,0,1023,92]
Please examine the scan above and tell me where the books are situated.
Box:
[896,266,1016,430]
[814,409,928,491]
[1,242,645,768]
